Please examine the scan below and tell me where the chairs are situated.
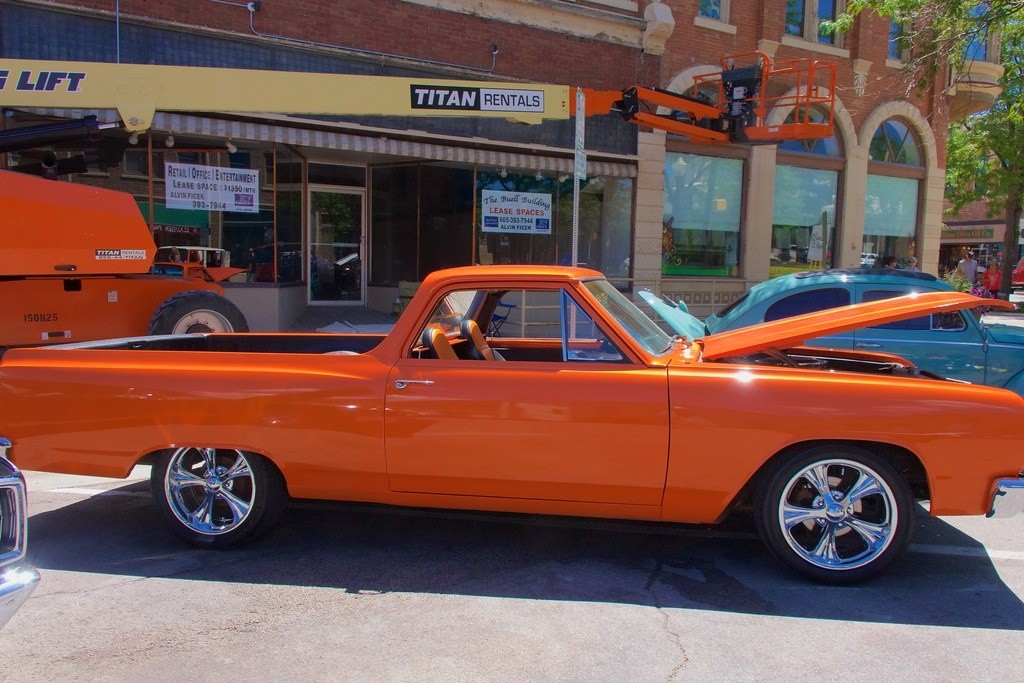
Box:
[421,327,459,360]
[433,309,464,329]
[485,301,517,338]
[460,319,506,360]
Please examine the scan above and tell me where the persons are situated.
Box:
[886,255,897,269]
[955,250,978,287]
[904,257,920,272]
[246,248,257,284]
[168,247,186,263]
[980,258,1003,299]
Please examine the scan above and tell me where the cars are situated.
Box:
[860,253,879,265]
[147,246,248,282]
[208,241,288,282]
[0,266,1023,588]
[638,267,1023,399]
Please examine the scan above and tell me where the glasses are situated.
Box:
[908,261,915,263]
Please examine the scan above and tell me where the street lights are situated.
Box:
[671,158,688,246]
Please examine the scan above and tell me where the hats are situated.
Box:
[966,251,975,258]
